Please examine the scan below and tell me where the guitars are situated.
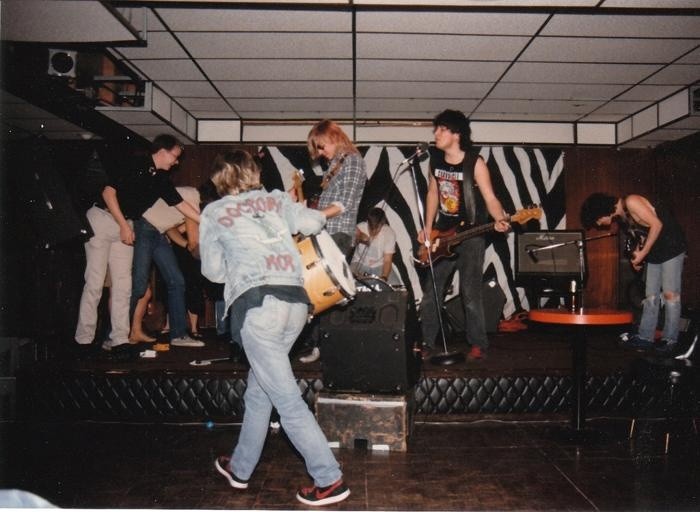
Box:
[625,237,644,271]
[418,203,542,267]
[292,171,307,201]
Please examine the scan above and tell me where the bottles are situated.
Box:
[569,281,579,313]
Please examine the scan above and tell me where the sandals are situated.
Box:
[192,332,203,337]
[130,332,158,342]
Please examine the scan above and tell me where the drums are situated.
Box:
[292,227,356,315]
[353,277,399,292]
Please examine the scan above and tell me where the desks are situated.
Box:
[529,308,633,434]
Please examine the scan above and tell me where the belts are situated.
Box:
[95,204,129,220]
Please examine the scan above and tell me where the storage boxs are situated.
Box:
[315,391,410,451]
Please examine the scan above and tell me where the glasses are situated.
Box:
[316,144,325,150]
[168,150,180,160]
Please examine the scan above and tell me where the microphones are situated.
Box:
[525,248,538,263]
[400,142,428,166]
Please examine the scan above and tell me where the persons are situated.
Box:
[307,121,367,264]
[416,109,512,366]
[353,208,396,288]
[74,133,217,351]
[581,193,688,348]
[199,148,351,507]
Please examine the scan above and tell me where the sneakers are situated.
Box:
[467,346,485,362]
[170,335,205,347]
[214,456,248,489]
[102,341,112,350]
[422,350,435,359]
[655,344,680,351]
[625,337,654,348]
[296,480,351,506]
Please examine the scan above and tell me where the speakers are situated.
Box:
[320,290,421,393]
